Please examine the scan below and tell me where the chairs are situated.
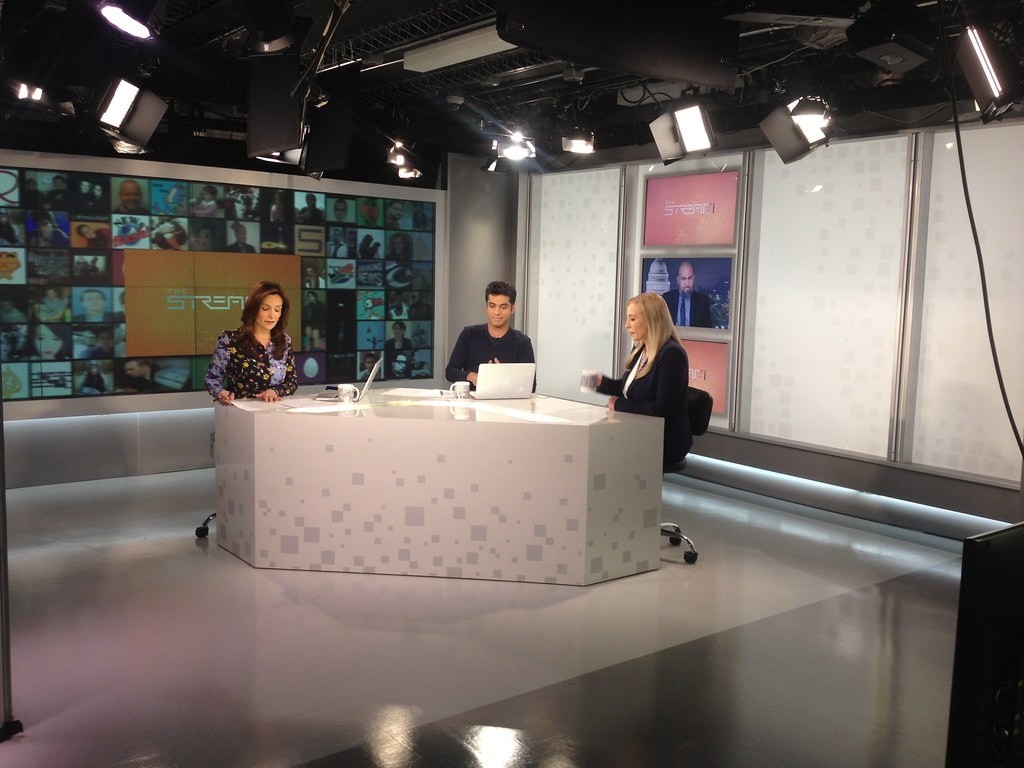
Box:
[195,512,218,537]
[660,386,712,564]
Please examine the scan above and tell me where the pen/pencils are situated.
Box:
[212,389,237,404]
[440,390,443,395]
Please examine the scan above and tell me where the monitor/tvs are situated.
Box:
[643,169,740,245]
[1,163,436,402]
[676,332,733,420]
[641,258,732,330]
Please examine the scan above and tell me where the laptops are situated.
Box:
[314,356,384,402]
[469,363,536,400]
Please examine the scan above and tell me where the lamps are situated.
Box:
[955,25,1015,123]
[17,83,75,118]
[480,158,509,175]
[97,71,169,154]
[387,132,423,181]
[758,93,832,164]
[491,128,537,160]
[649,101,720,166]
[561,126,593,154]
[94,0,160,42]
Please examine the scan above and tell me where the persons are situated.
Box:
[0,170,432,396]
[446,280,537,393]
[204,279,298,404]
[661,262,712,328]
[599,290,694,460]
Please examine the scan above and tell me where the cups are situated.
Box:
[338,383,360,403]
[450,380,471,399]
[579,369,599,393]
